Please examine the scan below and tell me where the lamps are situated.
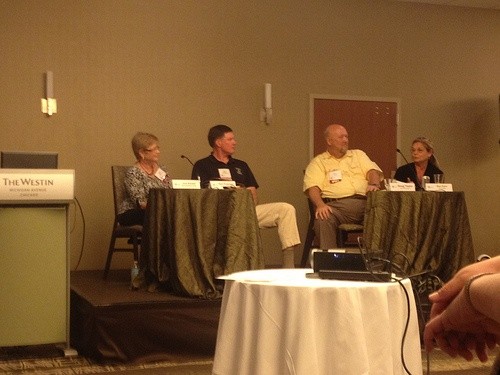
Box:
[258,82,272,124]
[41,71,57,115]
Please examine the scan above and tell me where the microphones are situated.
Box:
[396,149,423,191]
[181,155,201,179]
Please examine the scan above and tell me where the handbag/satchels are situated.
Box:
[128,260,164,293]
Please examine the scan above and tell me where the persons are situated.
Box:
[395,137,444,190]
[117,132,168,225]
[192,125,301,269]
[423,256,500,375]
[303,124,384,250]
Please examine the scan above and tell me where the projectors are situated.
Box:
[312,248,369,274]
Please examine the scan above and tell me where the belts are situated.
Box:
[325,194,367,202]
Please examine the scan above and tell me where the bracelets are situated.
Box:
[464,272,494,315]
[372,184,376,186]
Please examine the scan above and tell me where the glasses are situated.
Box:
[144,145,161,153]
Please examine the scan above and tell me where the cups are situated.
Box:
[433,174,445,183]
[383,178,395,191]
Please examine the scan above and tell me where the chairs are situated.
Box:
[105,165,149,279]
[300,170,364,267]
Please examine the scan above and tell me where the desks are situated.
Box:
[141,189,264,299]
[366,191,476,285]
[212,269,426,375]
